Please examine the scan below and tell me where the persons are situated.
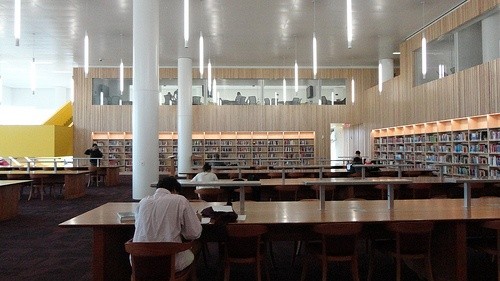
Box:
[84,143,104,181]
[130,175,202,275]
[374,129,500,177]
[190,153,226,199]
[351,151,362,162]
[235,92,243,101]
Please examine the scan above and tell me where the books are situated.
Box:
[94,140,314,173]
[116,210,135,221]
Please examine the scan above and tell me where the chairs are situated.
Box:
[103,94,332,105]
[0,165,500,281]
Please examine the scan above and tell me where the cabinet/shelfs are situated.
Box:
[370,112,500,177]
[91,130,316,170]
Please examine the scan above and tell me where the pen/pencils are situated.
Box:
[196,210,198,213]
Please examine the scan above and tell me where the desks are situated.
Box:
[179,166,440,177]
[176,177,500,199]
[0,163,124,187]
[56,197,500,281]
[0,179,34,221]
[194,164,393,169]
[0,170,91,199]
